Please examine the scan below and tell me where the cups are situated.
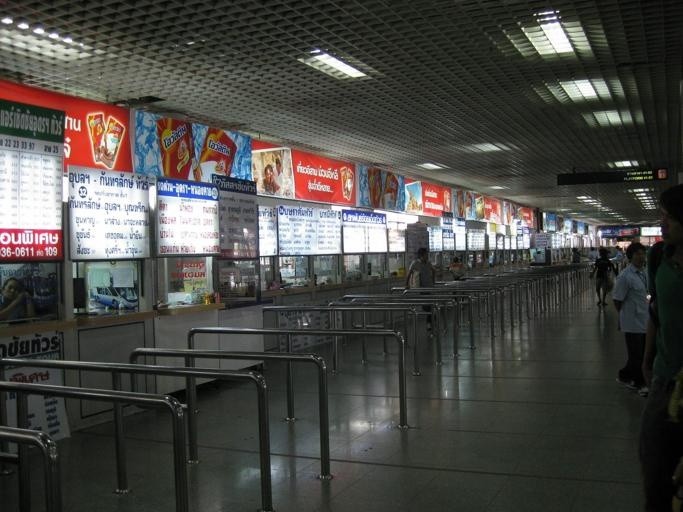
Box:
[204,289,210,304]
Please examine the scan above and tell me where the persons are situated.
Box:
[273,159,285,195]
[592,248,614,306]
[263,165,281,196]
[610,187,683,512]
[0,277,35,321]
[572,245,625,279]
[407,247,436,329]
[449,257,466,301]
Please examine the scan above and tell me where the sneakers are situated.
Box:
[616,377,637,389]
[638,386,649,397]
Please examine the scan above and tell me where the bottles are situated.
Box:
[119,301,124,315]
